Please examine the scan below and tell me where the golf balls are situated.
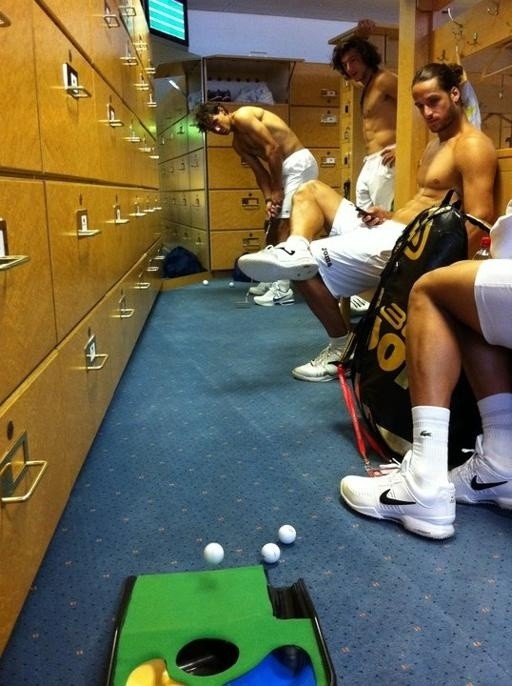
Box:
[203,280,209,286]
[279,524,298,544]
[229,282,234,287]
[262,543,281,563]
[204,542,225,564]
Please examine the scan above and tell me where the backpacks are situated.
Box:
[353,182,498,467]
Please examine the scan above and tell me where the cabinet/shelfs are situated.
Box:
[159,64,339,272]
[329,29,397,202]
[1,0,173,662]
[428,3,510,219]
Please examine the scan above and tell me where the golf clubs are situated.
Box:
[235,216,273,308]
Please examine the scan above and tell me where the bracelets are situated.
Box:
[264,197,272,204]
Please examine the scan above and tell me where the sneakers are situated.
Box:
[291,343,355,384]
[448,430,511,512]
[235,242,320,282]
[338,448,458,543]
[244,278,297,308]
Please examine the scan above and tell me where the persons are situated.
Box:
[328,34,398,323]
[237,60,500,389]
[339,188,512,542]
[193,98,320,309]
